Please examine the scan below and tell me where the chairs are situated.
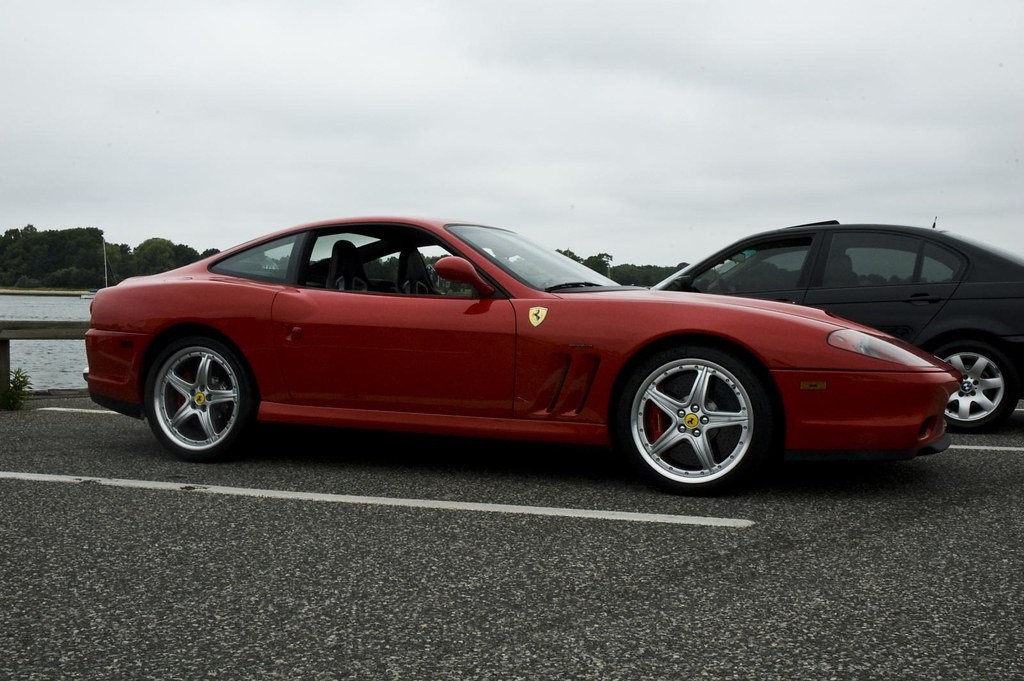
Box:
[823,252,856,290]
[325,241,440,294]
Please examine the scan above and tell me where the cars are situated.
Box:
[630,216,1024,434]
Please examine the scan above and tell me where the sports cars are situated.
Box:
[82,214,967,496]
